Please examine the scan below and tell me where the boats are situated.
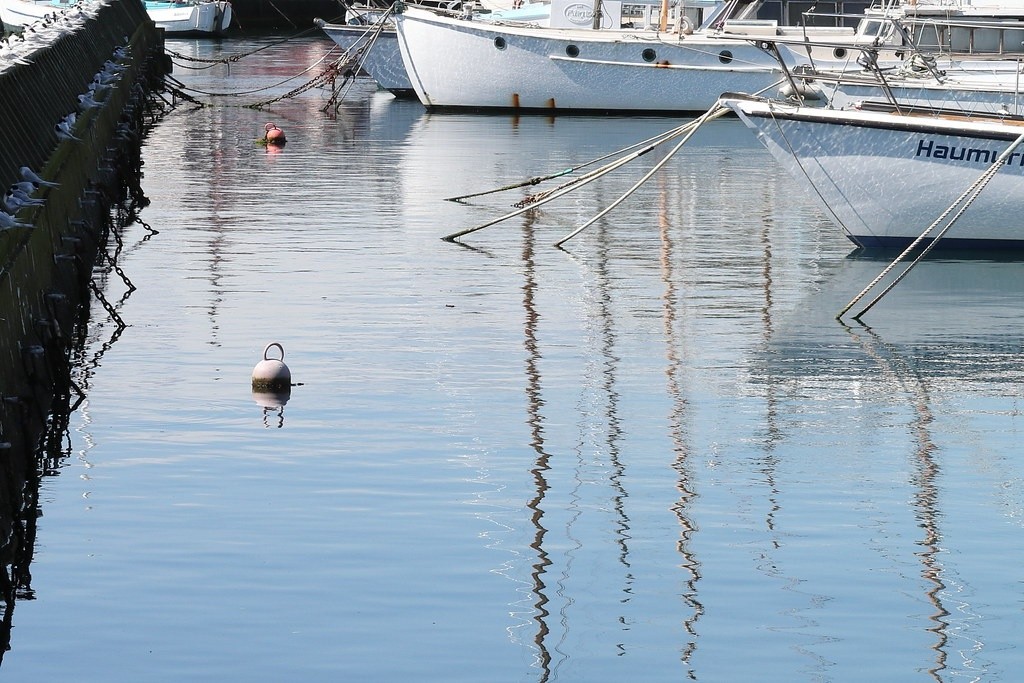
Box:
[313,0,1024,250]
[0,0,234,35]
